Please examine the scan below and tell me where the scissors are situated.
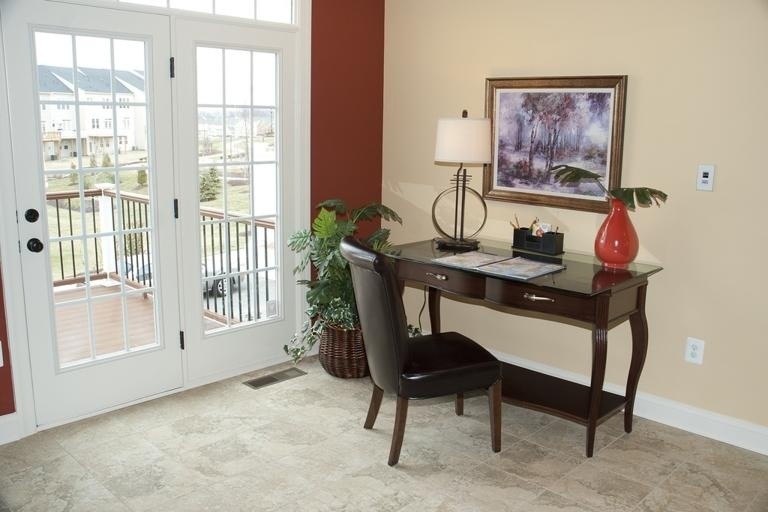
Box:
[536,230,544,238]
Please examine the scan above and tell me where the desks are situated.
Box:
[378,237,663,458]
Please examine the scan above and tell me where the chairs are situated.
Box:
[337,234,505,466]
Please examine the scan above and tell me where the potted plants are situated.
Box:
[276,197,405,378]
[548,161,668,270]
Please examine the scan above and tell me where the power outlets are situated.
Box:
[686,337,705,365]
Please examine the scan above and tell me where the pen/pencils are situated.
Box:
[555,227,558,235]
[510,212,520,230]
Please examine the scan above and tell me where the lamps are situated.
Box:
[431,108,494,254]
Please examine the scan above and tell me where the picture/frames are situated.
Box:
[481,74,628,216]
